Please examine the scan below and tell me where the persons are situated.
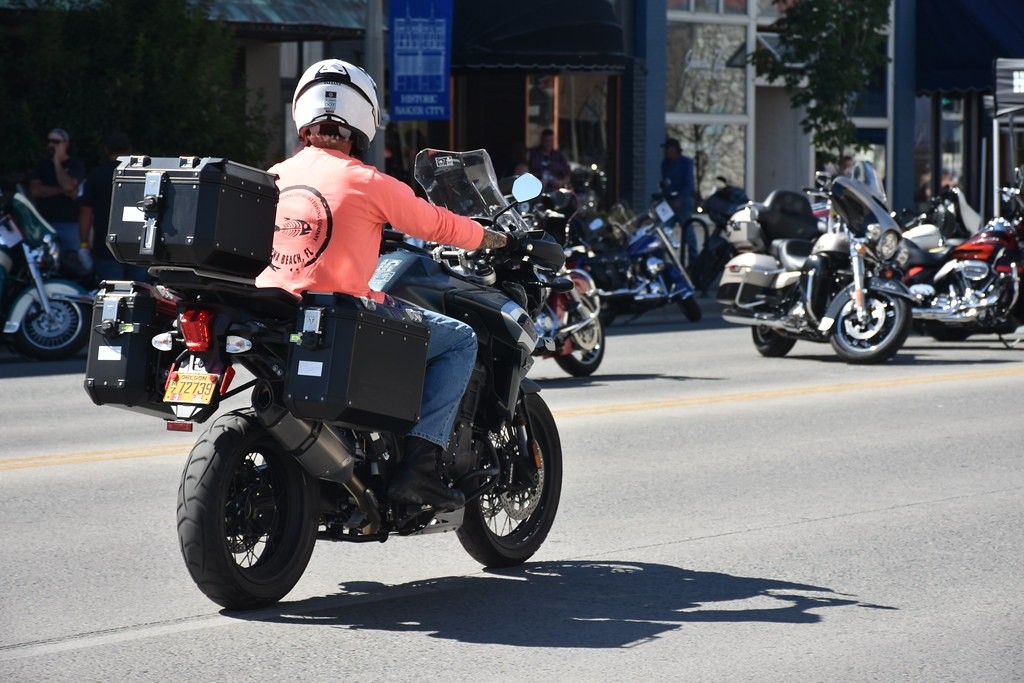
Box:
[254,59,535,510]
[29,129,154,283]
[658,138,698,269]
[512,128,575,191]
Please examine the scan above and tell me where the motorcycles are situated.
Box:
[84,149,577,616]
[1,160,1024,376]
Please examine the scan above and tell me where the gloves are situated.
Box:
[77,248,93,270]
[480,229,507,249]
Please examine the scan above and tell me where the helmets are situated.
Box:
[292,59,381,153]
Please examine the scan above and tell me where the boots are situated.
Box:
[388,437,465,512]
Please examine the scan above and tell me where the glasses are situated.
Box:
[45,138,68,144]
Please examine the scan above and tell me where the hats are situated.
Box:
[660,138,680,147]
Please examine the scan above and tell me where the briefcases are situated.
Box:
[105,154,279,279]
[85,280,219,424]
[282,289,431,435]
